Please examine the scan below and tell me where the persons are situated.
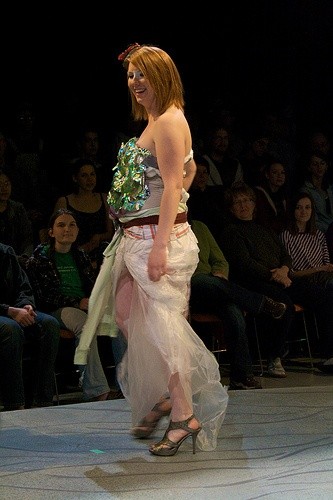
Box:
[217,181,293,379]
[73,43,229,456]
[53,159,115,266]
[187,157,230,245]
[0,243,61,411]
[56,127,110,194]
[199,124,242,186]
[0,132,33,211]
[273,192,333,372]
[186,208,286,390]
[252,136,282,184]
[253,161,296,229]
[302,131,333,174]
[0,170,35,275]
[298,150,333,263]
[26,209,127,401]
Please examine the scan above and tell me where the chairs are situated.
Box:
[51,293,324,405]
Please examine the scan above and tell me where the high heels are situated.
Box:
[129,398,171,439]
[149,413,201,456]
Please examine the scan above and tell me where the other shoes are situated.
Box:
[268,358,286,376]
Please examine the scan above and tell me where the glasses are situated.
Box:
[232,197,254,207]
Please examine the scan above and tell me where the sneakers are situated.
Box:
[230,372,262,389]
[262,296,287,318]
